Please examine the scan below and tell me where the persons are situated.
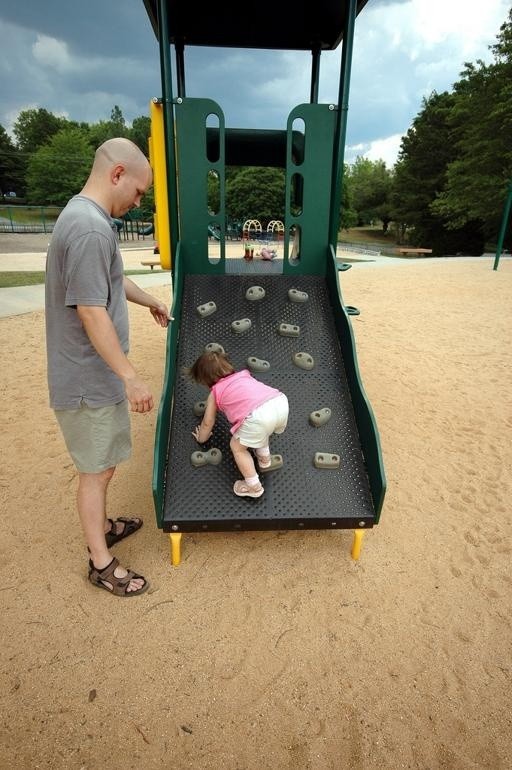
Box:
[45,137,175,597]
[189,350,289,498]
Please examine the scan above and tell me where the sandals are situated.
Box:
[87,515,144,554]
[87,556,149,597]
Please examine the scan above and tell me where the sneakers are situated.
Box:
[254,448,273,470]
[233,479,264,498]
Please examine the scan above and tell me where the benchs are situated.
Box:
[395,246,432,259]
[139,256,161,270]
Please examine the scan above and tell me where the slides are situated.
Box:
[114,218,154,235]
